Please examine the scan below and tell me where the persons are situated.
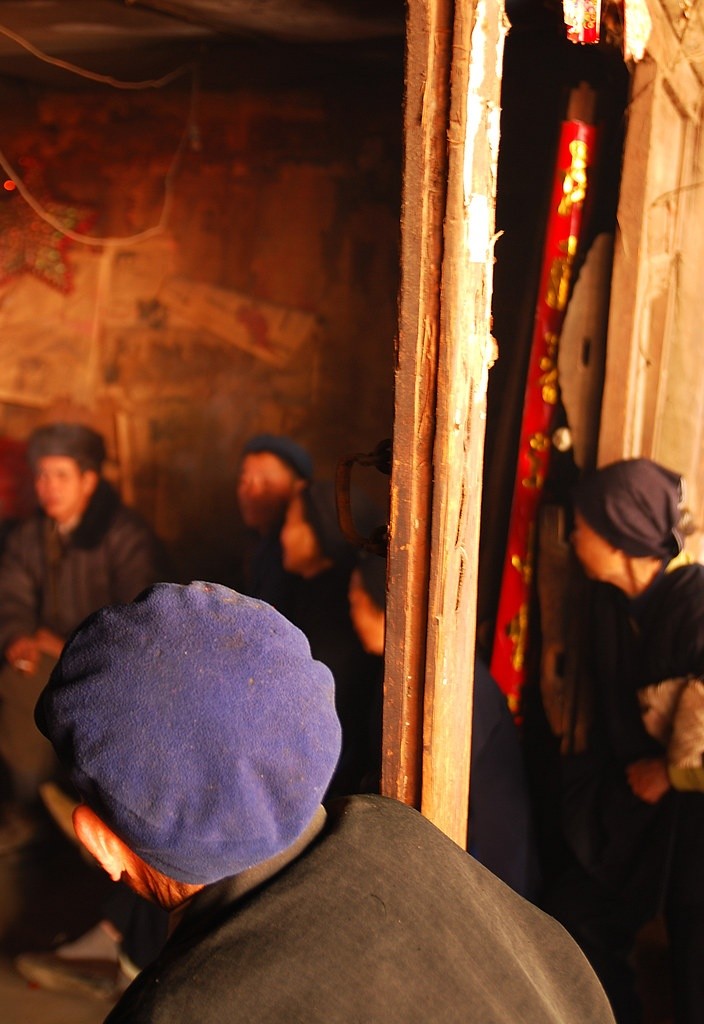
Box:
[35,581,612,1024]
[18,434,537,1000]
[569,457,704,1024]
[1,422,175,815]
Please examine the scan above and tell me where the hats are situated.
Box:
[243,434,310,482]
[27,421,107,471]
[35,579,342,884]
